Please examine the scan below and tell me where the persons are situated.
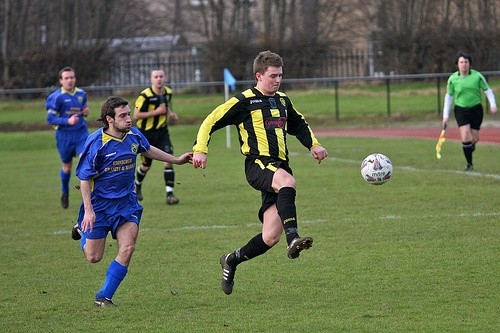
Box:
[441,51,498,171]
[71,96,193,308]
[46,67,90,208]
[192,50,329,296]
[132,70,179,206]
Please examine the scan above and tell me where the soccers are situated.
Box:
[360,153,393,185]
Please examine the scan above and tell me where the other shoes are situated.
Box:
[464,165,473,172]
[61,194,69,209]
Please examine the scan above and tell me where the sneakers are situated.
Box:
[71,225,82,240]
[287,236,314,260]
[166,195,179,206]
[135,183,143,201]
[94,297,118,308]
[219,253,235,296]
